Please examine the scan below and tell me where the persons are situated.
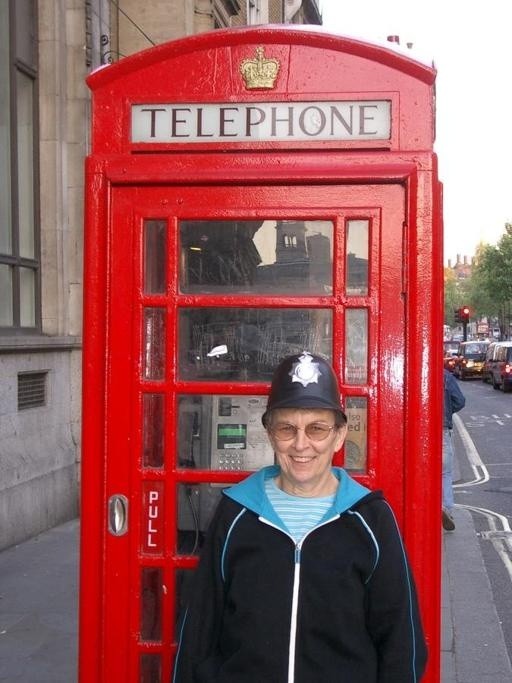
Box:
[172,351,427,683]
[442,368,465,531]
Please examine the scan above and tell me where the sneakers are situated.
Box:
[441,507,456,531]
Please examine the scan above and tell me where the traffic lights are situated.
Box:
[454,308,462,323]
[462,306,469,324]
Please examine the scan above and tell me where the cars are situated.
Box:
[187,321,361,390]
[443,325,512,393]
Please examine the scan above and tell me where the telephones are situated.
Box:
[177,411,200,486]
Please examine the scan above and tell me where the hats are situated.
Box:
[261,352,348,425]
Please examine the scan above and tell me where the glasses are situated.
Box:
[268,421,341,441]
